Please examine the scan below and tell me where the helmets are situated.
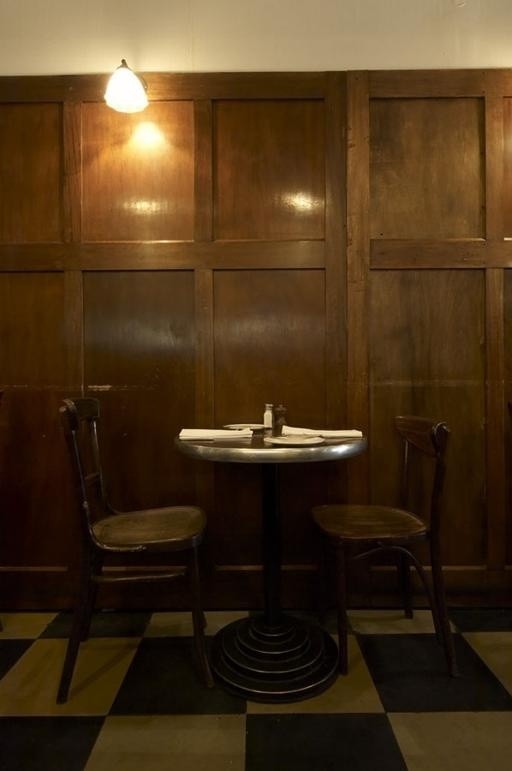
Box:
[263,434,326,445]
[224,423,264,430]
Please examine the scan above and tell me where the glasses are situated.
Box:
[104,59,148,114]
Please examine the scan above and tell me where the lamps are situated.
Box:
[55,396,214,705]
[308,415,458,676]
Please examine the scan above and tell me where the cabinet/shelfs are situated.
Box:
[263,403,288,437]
